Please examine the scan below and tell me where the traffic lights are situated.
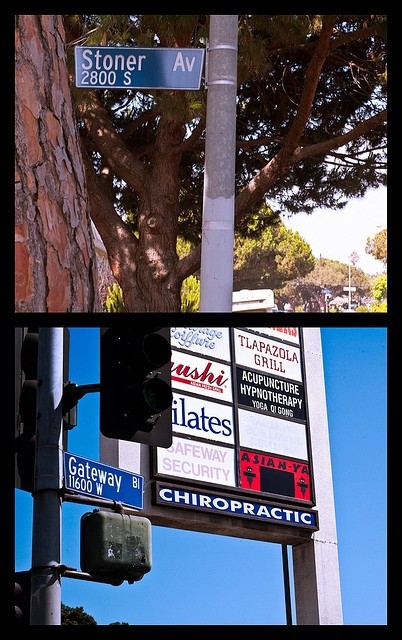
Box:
[99,324,173,449]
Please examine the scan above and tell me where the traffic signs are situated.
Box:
[75,47,205,91]
[62,451,144,511]
